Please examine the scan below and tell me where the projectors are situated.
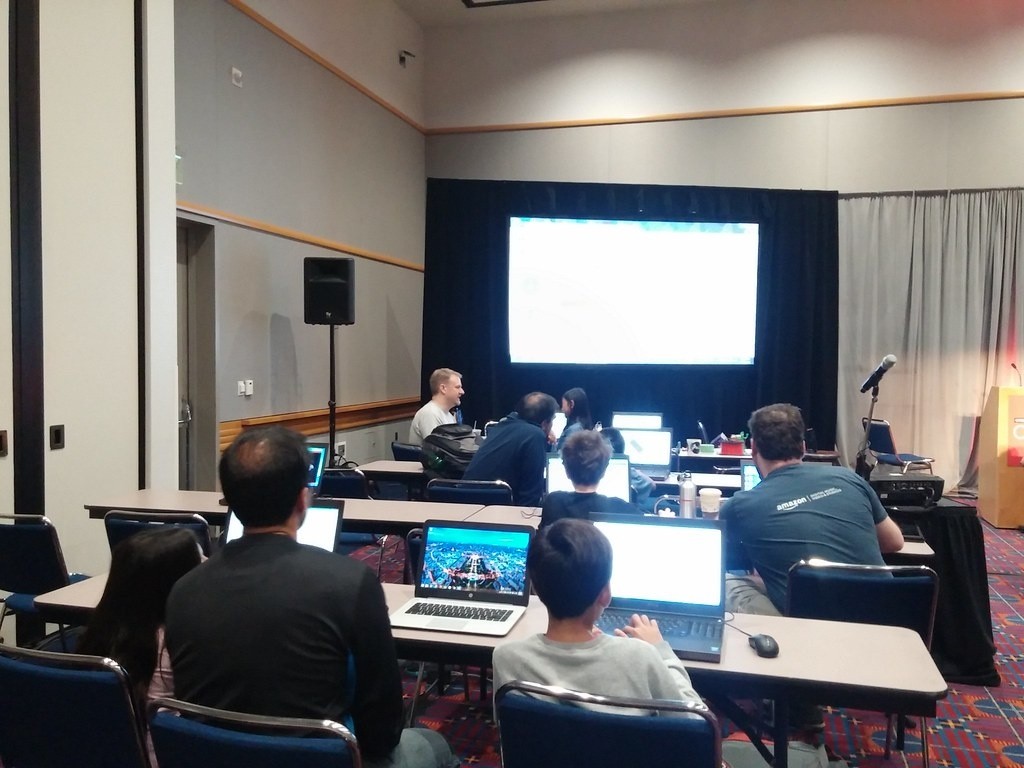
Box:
[869,471,945,506]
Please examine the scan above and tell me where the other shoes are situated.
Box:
[784,719,826,750]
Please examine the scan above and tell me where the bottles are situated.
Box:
[680,470,696,501]
[454,408,462,424]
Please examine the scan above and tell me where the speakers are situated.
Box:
[303,257,356,326]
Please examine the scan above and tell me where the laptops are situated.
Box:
[621,428,672,480]
[609,411,664,429]
[588,511,727,664]
[221,498,345,555]
[740,459,761,492]
[388,519,535,636]
[218,442,329,507]
[546,452,632,505]
[550,410,568,439]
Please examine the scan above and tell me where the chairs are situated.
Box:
[495,680,723,768]
[100,510,213,568]
[774,559,941,768]
[147,695,360,768]
[0,644,152,767]
[863,417,935,475]
[0,513,93,653]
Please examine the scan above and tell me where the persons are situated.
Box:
[81,528,209,768]
[408,368,465,446]
[718,403,906,619]
[461,386,658,515]
[493,518,719,723]
[163,427,460,768]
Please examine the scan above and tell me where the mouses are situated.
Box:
[748,634,779,658]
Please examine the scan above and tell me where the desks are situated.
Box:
[885,499,1001,686]
[362,458,742,490]
[35,567,948,768]
[85,489,942,545]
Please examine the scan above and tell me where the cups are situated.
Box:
[699,488,722,513]
[686,438,702,456]
[472,428,482,437]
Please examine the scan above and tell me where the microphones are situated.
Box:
[859,354,897,392]
[1011,363,1022,386]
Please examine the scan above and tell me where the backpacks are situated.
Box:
[420,423,487,482]
